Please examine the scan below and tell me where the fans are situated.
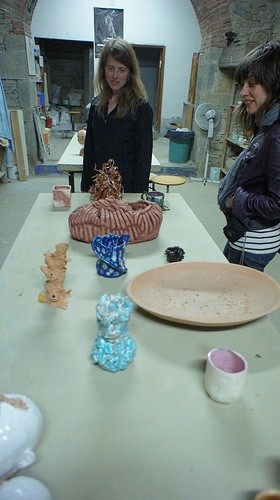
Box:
[190,102,220,185]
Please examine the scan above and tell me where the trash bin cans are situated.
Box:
[168,128,193,164]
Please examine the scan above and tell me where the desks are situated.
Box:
[0,131,280,500]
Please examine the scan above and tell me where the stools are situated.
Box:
[151,175,186,193]
[69,111,81,131]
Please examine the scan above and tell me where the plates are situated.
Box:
[126,262,280,327]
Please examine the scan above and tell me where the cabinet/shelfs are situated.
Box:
[219,70,252,176]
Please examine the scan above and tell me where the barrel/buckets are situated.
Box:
[8,166,18,179]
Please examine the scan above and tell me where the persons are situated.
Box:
[80,36,153,193]
[217,40,280,272]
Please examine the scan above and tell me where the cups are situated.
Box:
[91,233,130,279]
[205,348,248,404]
[141,191,165,210]
